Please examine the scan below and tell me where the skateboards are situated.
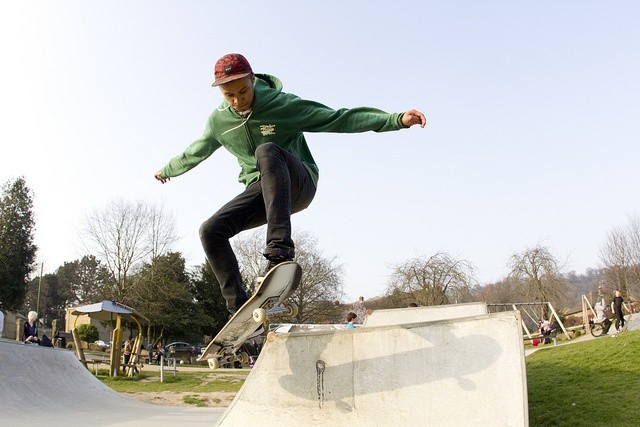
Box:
[195,261,302,369]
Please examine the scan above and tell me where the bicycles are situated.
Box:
[591,315,627,337]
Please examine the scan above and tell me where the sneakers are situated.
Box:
[258,260,285,283]
[247,324,265,339]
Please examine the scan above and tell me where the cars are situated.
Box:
[165,342,193,353]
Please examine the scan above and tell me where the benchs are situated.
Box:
[524,329,557,347]
[145,357,166,364]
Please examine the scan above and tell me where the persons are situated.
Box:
[593,296,612,336]
[346,312,357,328]
[154,344,165,364]
[153,52,427,339]
[540,314,552,344]
[24,311,38,344]
[124,336,139,374]
[141,344,153,365]
[407,303,417,307]
[611,289,624,330]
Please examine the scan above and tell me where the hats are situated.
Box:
[212,53,252,87]
